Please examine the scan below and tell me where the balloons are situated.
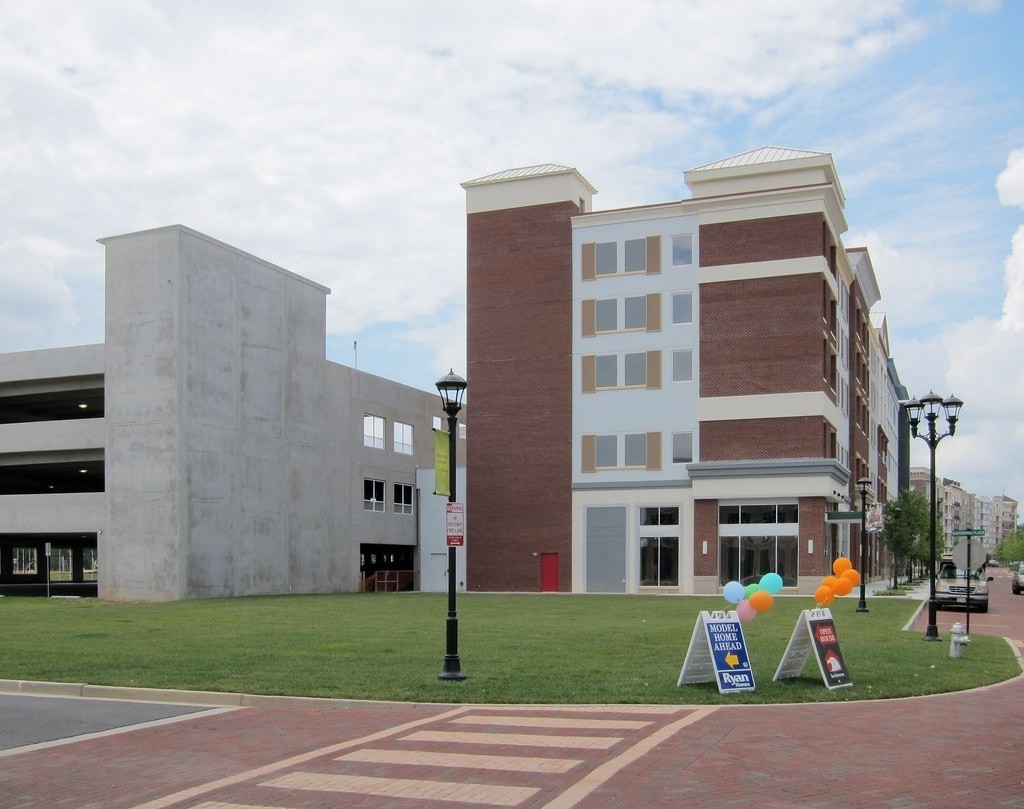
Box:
[815,558,860,605]
[723,573,782,621]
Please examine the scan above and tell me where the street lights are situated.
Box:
[435,368,469,684]
[903,389,964,641]
[855,477,872,613]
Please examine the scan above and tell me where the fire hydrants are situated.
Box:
[949,620,971,659]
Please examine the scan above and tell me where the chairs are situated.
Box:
[947,571,956,578]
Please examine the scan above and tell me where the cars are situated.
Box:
[935,553,994,613]
[1011,565,1024,595]
[988,560,1000,568]
